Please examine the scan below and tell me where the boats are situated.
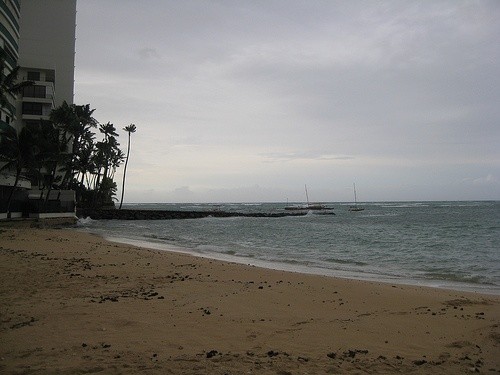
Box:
[284,185,335,214]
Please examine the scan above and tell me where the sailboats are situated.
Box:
[349,184,364,212]
